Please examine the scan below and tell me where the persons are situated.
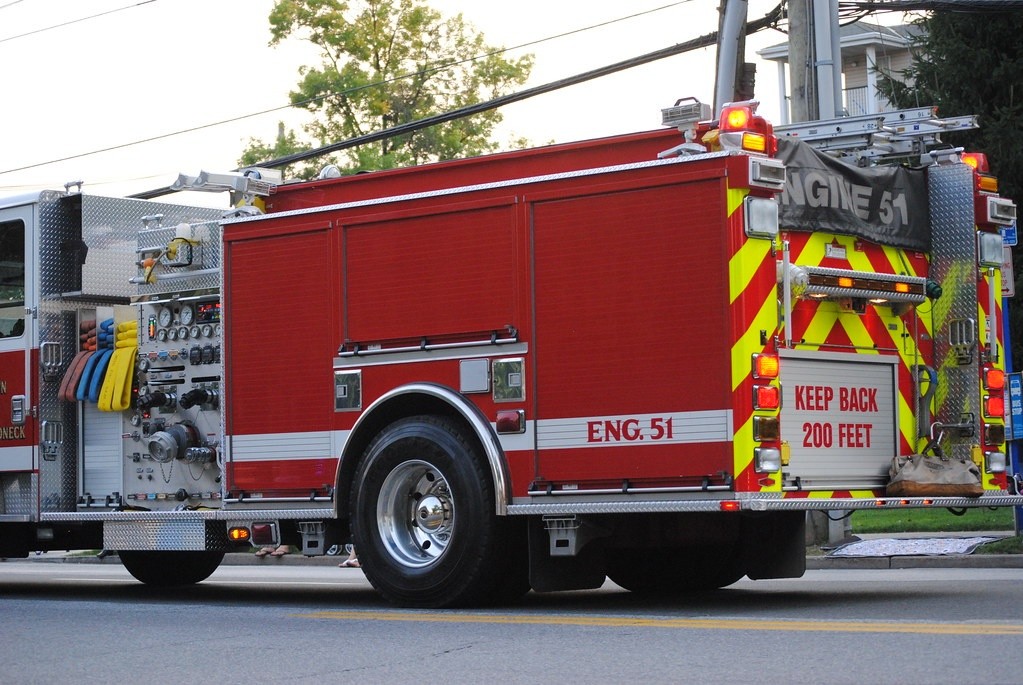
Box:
[254,543,293,558]
[339,542,364,568]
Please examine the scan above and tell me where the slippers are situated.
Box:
[270,549,292,556]
[254,549,269,557]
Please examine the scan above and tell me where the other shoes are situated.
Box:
[338,558,350,567]
[347,559,360,567]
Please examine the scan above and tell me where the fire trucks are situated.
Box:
[0,96,1022,611]
